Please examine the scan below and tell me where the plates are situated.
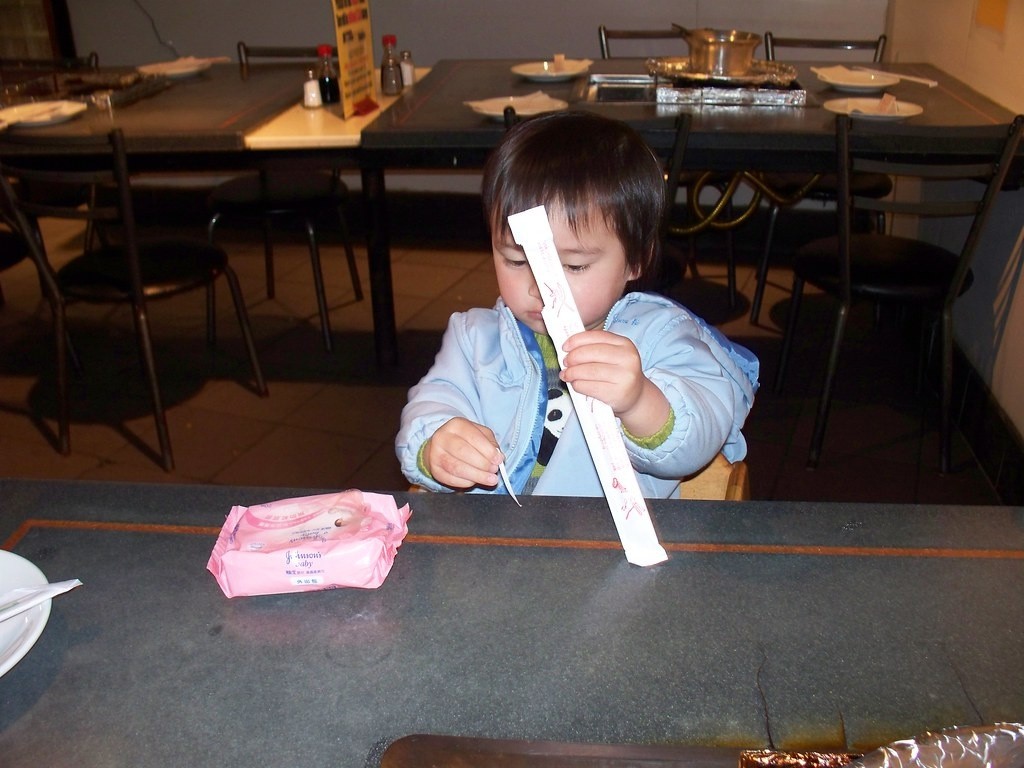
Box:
[138,59,210,76]
[510,60,588,82]
[824,98,926,119]
[0,101,88,125]
[818,68,903,92]
[645,57,797,84]
[0,548,53,677]
[473,96,568,121]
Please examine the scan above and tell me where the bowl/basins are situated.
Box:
[684,28,762,74]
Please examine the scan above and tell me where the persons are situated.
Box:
[393,112,760,500]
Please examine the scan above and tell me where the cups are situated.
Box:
[555,54,566,74]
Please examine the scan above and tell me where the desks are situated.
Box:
[0,478,1024,768]
[361,60,1024,382]
[0,60,428,390]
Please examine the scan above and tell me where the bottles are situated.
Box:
[397,50,416,86]
[315,45,340,104]
[304,69,322,107]
[381,35,401,95]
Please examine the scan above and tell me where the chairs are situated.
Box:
[0,26,1022,504]
